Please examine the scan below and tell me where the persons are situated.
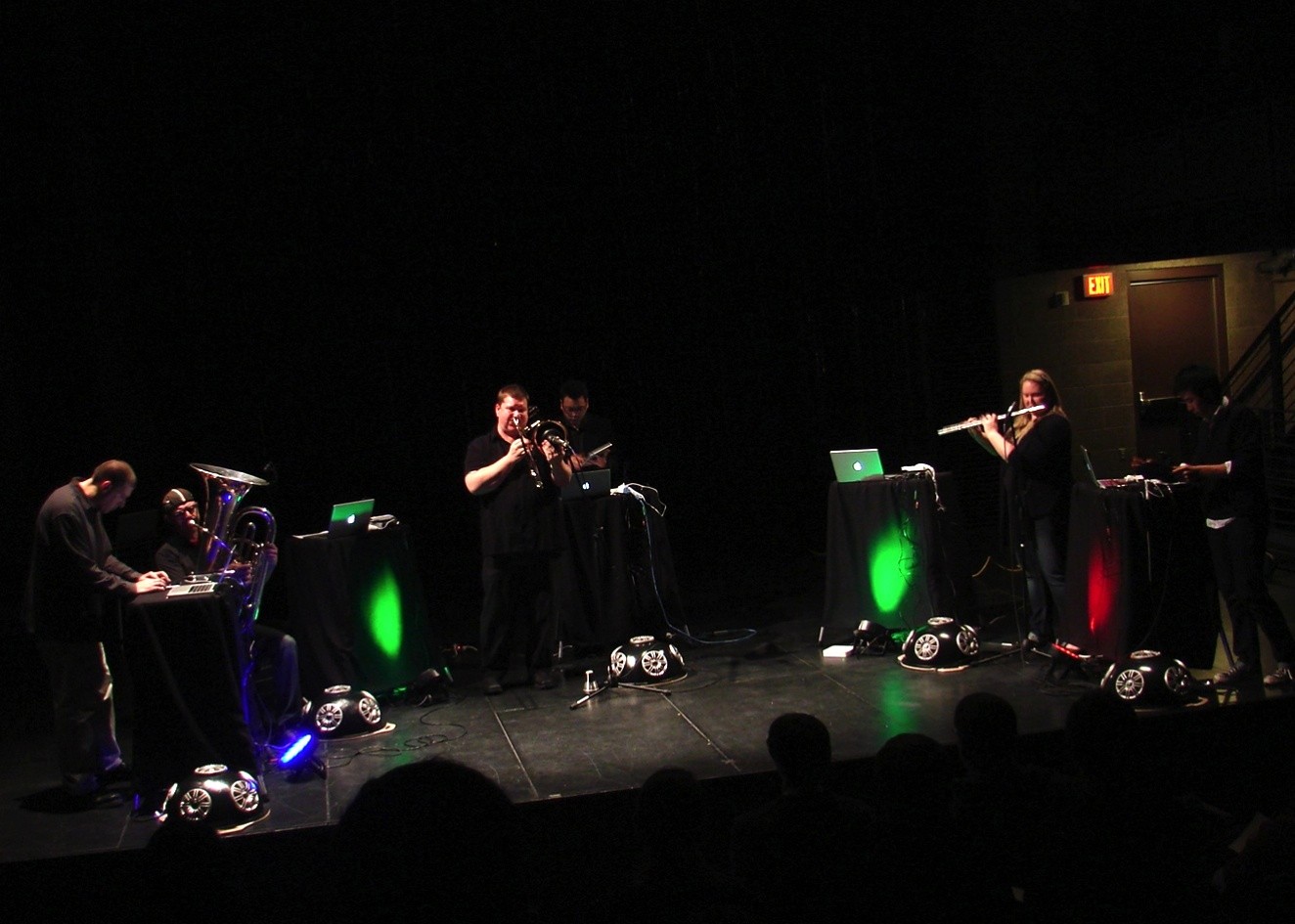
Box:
[543,381,611,502]
[155,487,319,798]
[29,459,172,809]
[1117,362,1295,685]
[463,384,574,694]
[962,369,1073,659]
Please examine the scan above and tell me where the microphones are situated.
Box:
[1003,402,1017,425]
[548,435,569,447]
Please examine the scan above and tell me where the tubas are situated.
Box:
[187,458,279,630]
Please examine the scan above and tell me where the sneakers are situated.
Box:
[1263,668,1295,686]
[1213,661,1262,686]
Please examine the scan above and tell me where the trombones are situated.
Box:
[514,418,569,490]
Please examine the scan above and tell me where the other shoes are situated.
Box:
[1021,633,1058,648]
[483,675,504,694]
[70,787,124,808]
[97,766,155,800]
[536,678,560,690]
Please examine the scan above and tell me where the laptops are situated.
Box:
[304,499,375,541]
[1079,444,1126,490]
[166,544,237,596]
[829,448,902,482]
[559,469,610,502]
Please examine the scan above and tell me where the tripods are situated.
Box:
[568,455,674,710]
[969,425,1096,670]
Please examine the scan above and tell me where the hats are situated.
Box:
[162,488,194,514]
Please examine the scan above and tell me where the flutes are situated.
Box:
[935,403,1048,435]
